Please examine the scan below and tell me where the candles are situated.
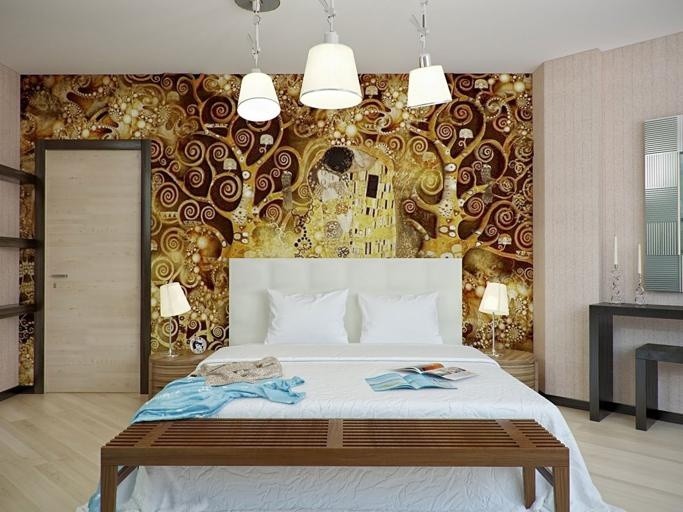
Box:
[614,236,618,265]
[638,244,641,274]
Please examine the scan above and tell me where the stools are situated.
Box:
[635,343,683,432]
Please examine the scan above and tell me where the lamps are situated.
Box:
[160,282,191,358]
[233,0,281,123]
[298,0,363,110]
[478,282,509,358]
[406,0,453,109]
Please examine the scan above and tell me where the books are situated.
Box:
[391,362,479,381]
[363,371,457,392]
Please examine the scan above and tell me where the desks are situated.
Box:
[589,301,683,422]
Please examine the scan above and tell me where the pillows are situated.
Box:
[358,292,442,344]
[263,287,349,347]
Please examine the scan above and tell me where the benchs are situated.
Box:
[99,417,571,510]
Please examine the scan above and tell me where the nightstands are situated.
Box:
[478,349,539,394]
[149,350,214,400]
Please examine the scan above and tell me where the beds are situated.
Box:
[86,257,624,512]
